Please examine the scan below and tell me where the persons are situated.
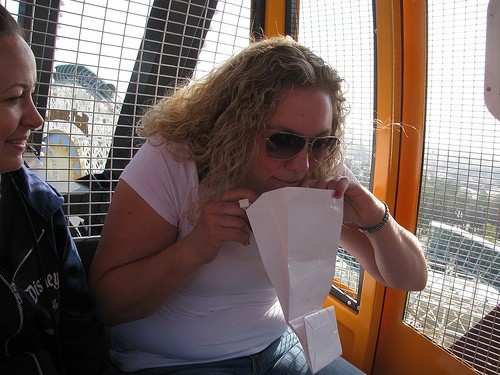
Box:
[87,35,427,375]
[0,4,87,375]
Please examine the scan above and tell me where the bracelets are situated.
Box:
[358,201,389,234]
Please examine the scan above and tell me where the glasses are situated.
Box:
[259,132,340,163]
